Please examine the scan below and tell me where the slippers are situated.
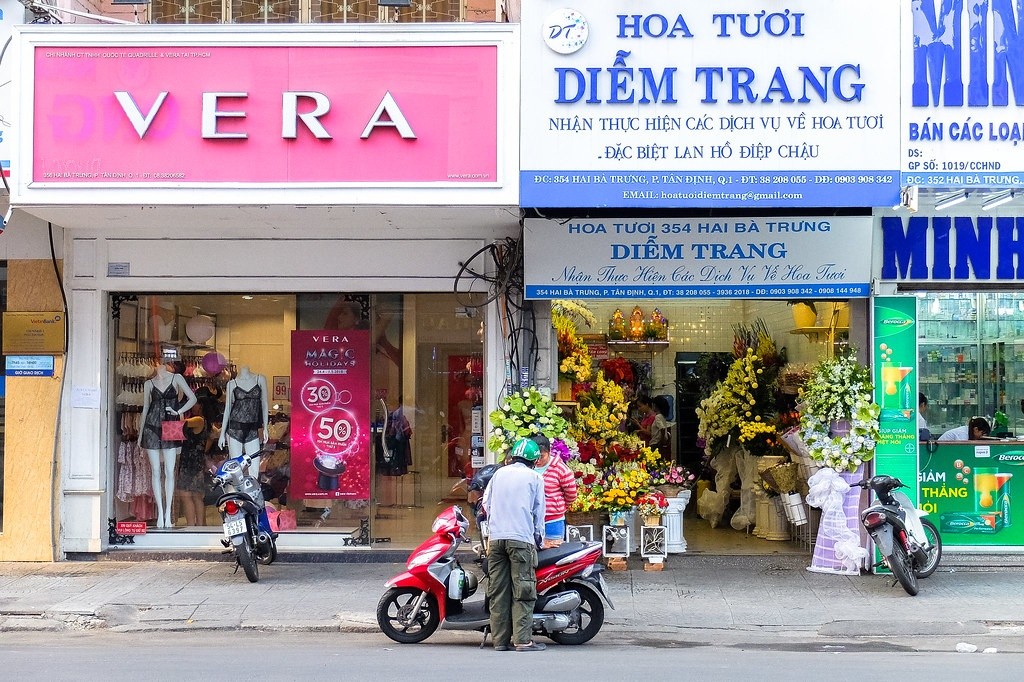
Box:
[495,642,516,651]
[515,640,546,651]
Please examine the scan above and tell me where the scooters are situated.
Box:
[467,461,506,584]
[376,504,615,650]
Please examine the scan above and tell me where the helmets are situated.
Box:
[464,570,478,599]
[511,438,541,460]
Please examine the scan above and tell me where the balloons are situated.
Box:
[202,353,226,375]
[186,316,215,344]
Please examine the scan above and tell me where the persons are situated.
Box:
[324,295,399,366]
[614,311,662,334]
[919,392,928,428]
[468,439,564,651]
[218,366,268,479]
[621,381,670,454]
[176,388,220,525]
[138,365,197,527]
[938,418,991,440]
[534,432,577,540]
[456,370,477,476]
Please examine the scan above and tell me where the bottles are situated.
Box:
[448,566,465,600]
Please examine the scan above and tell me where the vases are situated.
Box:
[658,484,680,498]
[793,302,816,328]
[608,512,626,526]
[643,515,660,526]
[565,510,593,525]
[747,493,808,541]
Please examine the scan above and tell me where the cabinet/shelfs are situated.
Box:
[916,291,1024,436]
[117,350,237,523]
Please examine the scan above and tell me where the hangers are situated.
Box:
[120,352,202,372]
[122,375,151,394]
[185,376,213,389]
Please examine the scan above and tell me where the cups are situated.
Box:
[973,467,1012,528]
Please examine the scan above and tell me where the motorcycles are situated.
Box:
[203,449,279,583]
[849,474,942,597]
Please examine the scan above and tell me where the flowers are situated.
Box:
[693,316,882,498]
[488,301,696,511]
